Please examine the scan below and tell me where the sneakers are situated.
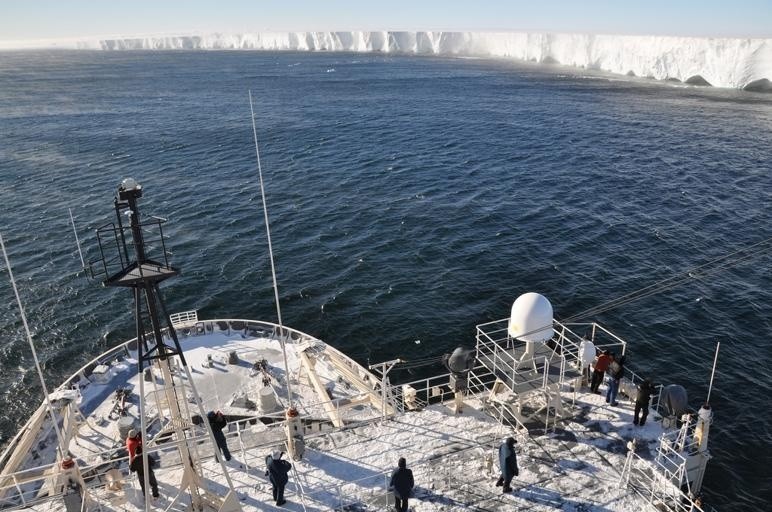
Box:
[282,500,286,504]
[496,483,512,492]
[154,493,159,500]
[595,391,618,406]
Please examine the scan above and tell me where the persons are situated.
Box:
[590,350,612,394]
[129,446,159,497]
[126,430,142,463]
[606,355,626,406]
[496,437,519,493]
[266,450,291,506]
[207,411,231,462]
[633,377,658,426]
[390,458,414,512]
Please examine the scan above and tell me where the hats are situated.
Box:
[128,429,137,438]
[398,458,406,467]
[208,412,214,417]
[136,447,142,454]
[272,450,281,460]
[507,438,517,445]
